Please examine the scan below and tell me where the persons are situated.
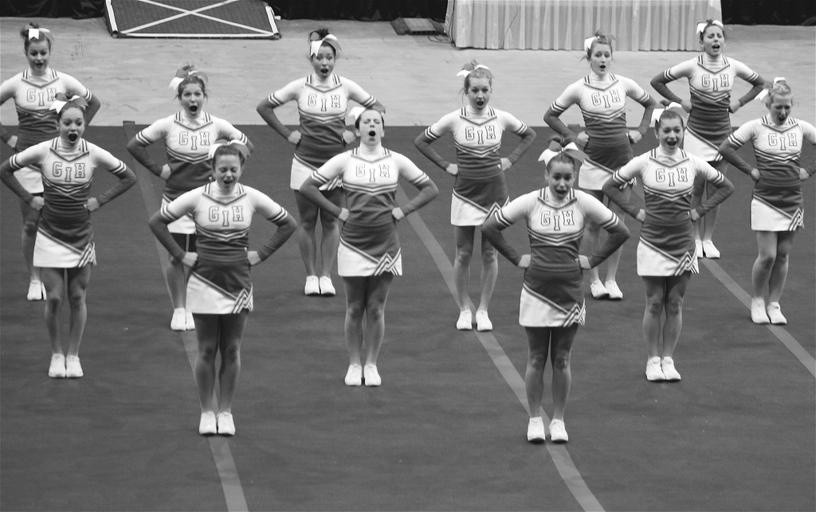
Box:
[544,35,654,300]
[0,97,138,378]
[299,110,439,388]
[0,23,101,302]
[256,32,387,296]
[604,101,734,383]
[415,61,536,331]
[650,19,764,259]
[126,66,254,333]
[483,136,631,443]
[148,141,297,434]
[719,76,816,325]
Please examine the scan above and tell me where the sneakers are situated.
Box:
[26,279,47,299]
[49,353,84,379]
[198,411,217,436]
[456,309,474,331]
[750,297,787,325]
[167,305,195,330]
[364,365,382,386]
[476,311,494,331]
[217,412,236,437]
[551,419,570,443]
[695,237,720,258]
[527,417,544,443]
[605,281,624,301]
[645,354,682,382]
[345,365,363,388]
[589,279,609,300]
[301,275,335,297]
